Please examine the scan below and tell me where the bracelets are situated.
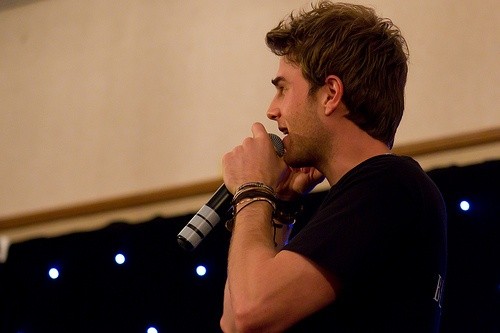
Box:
[230,181,277,217]
[272,198,304,228]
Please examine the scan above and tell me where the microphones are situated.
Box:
[176,133,283,250]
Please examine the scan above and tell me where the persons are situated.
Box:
[220,1,450,333]
